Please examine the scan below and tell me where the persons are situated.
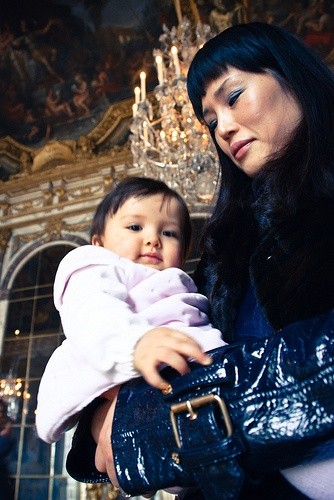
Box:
[36,175,227,448]
[67,20,334,499]
[2,414,16,497]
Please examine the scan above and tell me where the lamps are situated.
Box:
[0,368,23,420]
[127,0,224,207]
